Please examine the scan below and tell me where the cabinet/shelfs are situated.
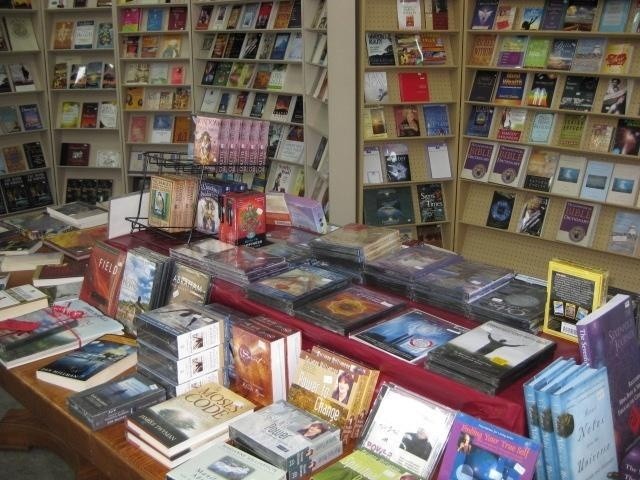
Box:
[302,0,330,224]
[356,0,464,253]
[1,0,58,215]
[460,1,640,294]
[113,2,194,193]
[41,0,124,205]
[190,0,304,199]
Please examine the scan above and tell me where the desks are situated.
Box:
[1,199,639,480]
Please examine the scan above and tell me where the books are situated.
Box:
[0,0,640,480]
